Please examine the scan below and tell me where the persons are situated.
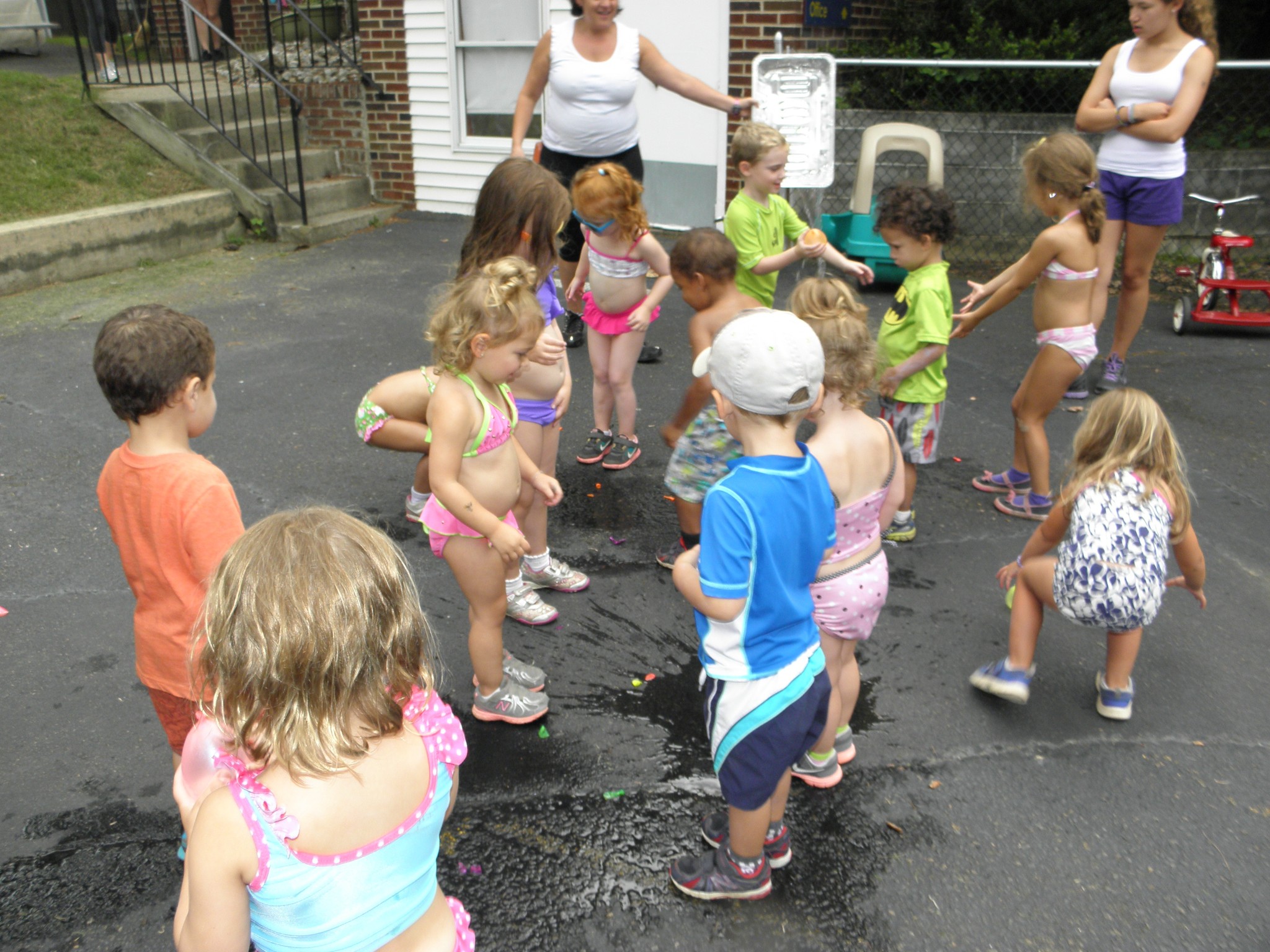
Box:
[871,181,959,542]
[1062,0,1220,399]
[454,157,591,626]
[946,132,1107,522]
[723,121,875,309]
[786,277,906,788]
[92,303,246,861]
[655,226,764,570]
[354,365,440,522]
[968,386,1208,720]
[509,0,759,361]
[171,503,475,952]
[668,306,832,900]
[417,254,564,724]
[81,0,118,83]
[190,0,226,62]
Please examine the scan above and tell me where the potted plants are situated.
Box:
[297,0,343,42]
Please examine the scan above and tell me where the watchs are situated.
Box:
[731,97,741,116]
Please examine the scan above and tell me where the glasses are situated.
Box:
[572,209,617,235]
[519,221,566,244]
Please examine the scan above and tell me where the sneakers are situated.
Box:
[471,676,549,724]
[405,496,431,523]
[520,557,589,592]
[833,727,856,765]
[879,503,917,541]
[1095,665,1133,720]
[505,583,559,625]
[577,427,614,463]
[638,342,662,364]
[700,811,793,869]
[473,648,548,692]
[655,537,689,570]
[561,311,584,347]
[1064,369,1090,399]
[1096,352,1125,390]
[791,748,842,788]
[601,433,641,468]
[669,839,772,900]
[969,657,1035,704]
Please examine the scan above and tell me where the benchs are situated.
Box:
[0,23,62,56]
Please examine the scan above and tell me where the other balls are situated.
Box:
[805,229,828,250]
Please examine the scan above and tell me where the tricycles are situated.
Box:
[1173,192,1270,333]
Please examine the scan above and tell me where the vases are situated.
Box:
[269,12,299,43]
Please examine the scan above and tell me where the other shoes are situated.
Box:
[994,489,1053,521]
[972,470,1032,494]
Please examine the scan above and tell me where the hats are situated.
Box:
[692,307,825,415]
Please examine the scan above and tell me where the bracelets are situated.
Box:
[1115,106,1128,126]
[1017,555,1024,568]
[1128,104,1136,124]
